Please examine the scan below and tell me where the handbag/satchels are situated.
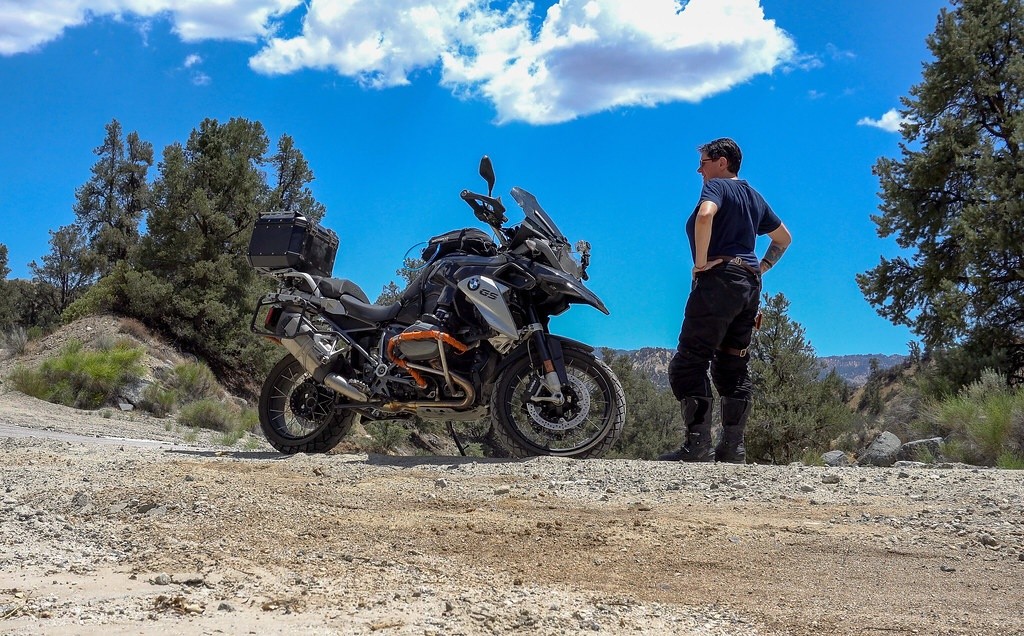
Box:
[422,228,496,264]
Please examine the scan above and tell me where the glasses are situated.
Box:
[700,157,720,167]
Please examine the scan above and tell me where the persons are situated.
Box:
[667,137,792,464]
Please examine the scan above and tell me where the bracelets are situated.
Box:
[761,258,774,268]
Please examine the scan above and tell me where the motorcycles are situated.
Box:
[245,155,626,460]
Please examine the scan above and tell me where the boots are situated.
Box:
[656,396,716,462]
[715,396,754,465]
